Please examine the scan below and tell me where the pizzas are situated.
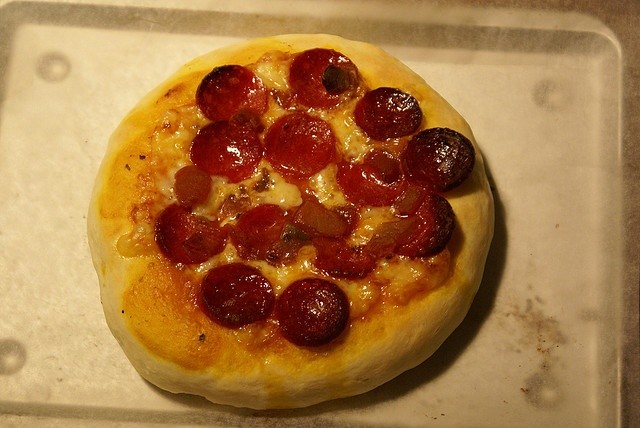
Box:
[83,31,499,414]
